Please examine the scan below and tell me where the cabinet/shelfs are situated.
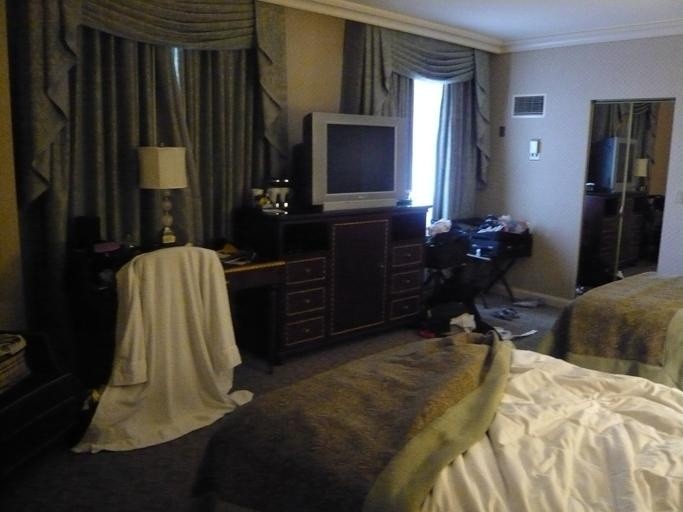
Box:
[232,198,435,365]
[578,189,648,279]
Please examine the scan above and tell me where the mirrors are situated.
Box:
[572,97,678,299]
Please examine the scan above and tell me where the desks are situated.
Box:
[66,242,286,428]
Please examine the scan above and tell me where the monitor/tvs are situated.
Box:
[292,110,400,211]
[596,137,637,193]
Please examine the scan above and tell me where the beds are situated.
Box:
[534,272,681,390]
[187,334,681,512]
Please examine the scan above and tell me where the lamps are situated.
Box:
[633,158,650,193]
